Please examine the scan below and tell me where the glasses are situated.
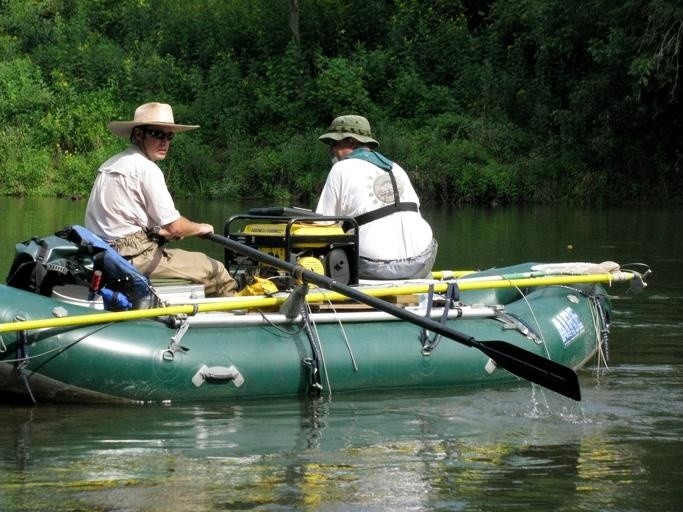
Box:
[142,127,176,140]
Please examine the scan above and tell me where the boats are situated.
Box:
[0,262,610,407]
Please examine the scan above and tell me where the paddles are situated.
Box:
[201,232,582,402]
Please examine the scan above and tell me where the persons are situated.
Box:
[314,114,437,280]
[84,101,239,298]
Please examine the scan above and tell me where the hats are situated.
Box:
[318,114,380,149]
[108,102,200,138]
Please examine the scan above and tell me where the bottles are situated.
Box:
[99,288,134,309]
